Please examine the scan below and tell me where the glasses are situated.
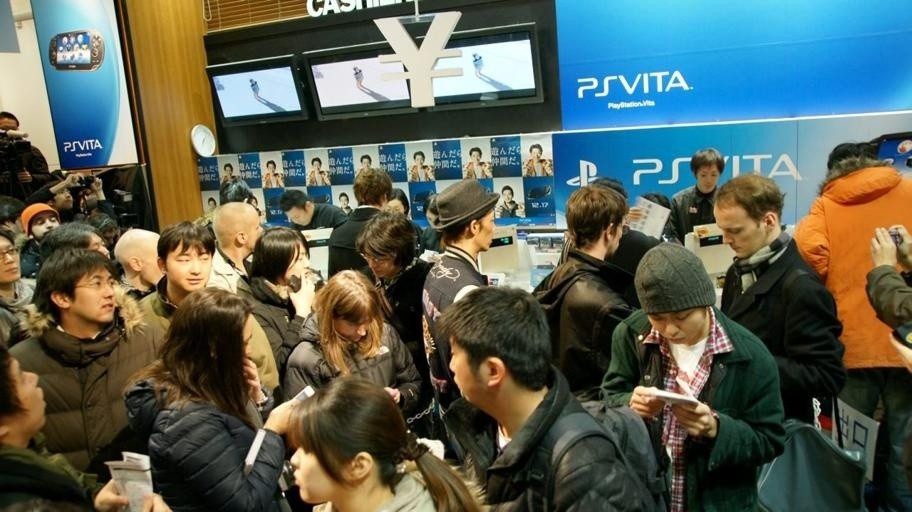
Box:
[360,252,385,263]
[0,247,20,260]
[78,279,116,288]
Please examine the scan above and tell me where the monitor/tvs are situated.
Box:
[415,22,544,113]
[301,40,420,122]
[205,54,310,128]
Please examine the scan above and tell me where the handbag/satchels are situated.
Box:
[754,418,867,512]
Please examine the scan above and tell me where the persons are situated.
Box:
[409,150,435,183]
[522,143,552,177]
[711,171,853,423]
[864,224,911,376]
[793,142,912,512]
[661,149,725,248]
[1,143,305,512]
[284,164,790,512]
[463,147,493,180]
[306,158,332,188]
[359,154,372,169]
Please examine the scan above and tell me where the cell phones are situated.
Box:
[289,275,301,292]
[292,384,315,403]
[653,389,697,409]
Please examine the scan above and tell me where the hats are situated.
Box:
[430,178,499,235]
[20,203,61,237]
[635,243,715,315]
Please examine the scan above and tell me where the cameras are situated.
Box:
[79,175,94,187]
[888,231,903,247]
